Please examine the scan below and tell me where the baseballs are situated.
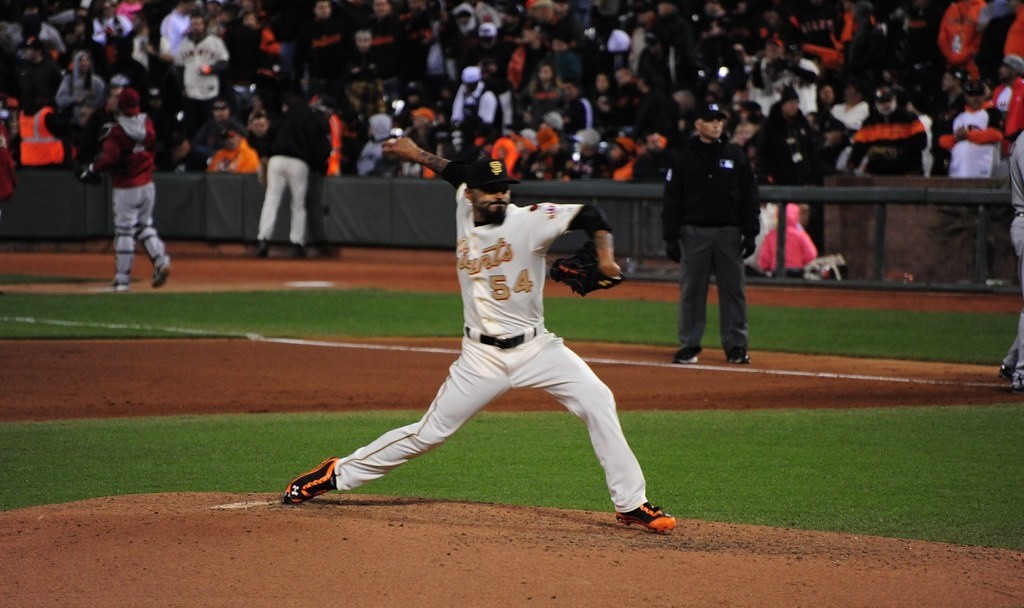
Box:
[384,138,399,159]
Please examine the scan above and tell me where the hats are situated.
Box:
[615,137,638,154]
[411,108,434,122]
[779,87,798,103]
[536,127,556,152]
[694,100,727,121]
[478,21,497,38]
[118,88,141,115]
[462,67,481,82]
[466,157,519,187]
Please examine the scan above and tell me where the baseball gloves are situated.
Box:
[548,240,629,297]
[75,163,103,186]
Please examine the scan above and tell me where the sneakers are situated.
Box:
[284,457,340,505]
[616,503,676,532]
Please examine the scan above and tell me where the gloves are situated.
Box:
[666,241,682,263]
[740,237,756,258]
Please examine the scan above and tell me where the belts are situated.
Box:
[465,327,535,349]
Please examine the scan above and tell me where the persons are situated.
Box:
[598,0,1024,178]
[77,88,171,289]
[997,132,1024,387]
[246,98,337,260]
[661,104,761,365]
[0,120,19,200]
[744,203,846,278]
[283,136,676,534]
[0,0,613,181]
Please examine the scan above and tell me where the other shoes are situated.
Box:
[998,365,1015,381]
[289,244,305,259]
[673,346,698,363]
[1013,377,1024,393]
[256,240,268,259]
[728,348,750,363]
[113,284,128,292]
[151,264,169,288]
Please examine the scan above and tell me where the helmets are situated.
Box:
[963,79,987,100]
[872,86,892,103]
[1003,54,1024,73]
